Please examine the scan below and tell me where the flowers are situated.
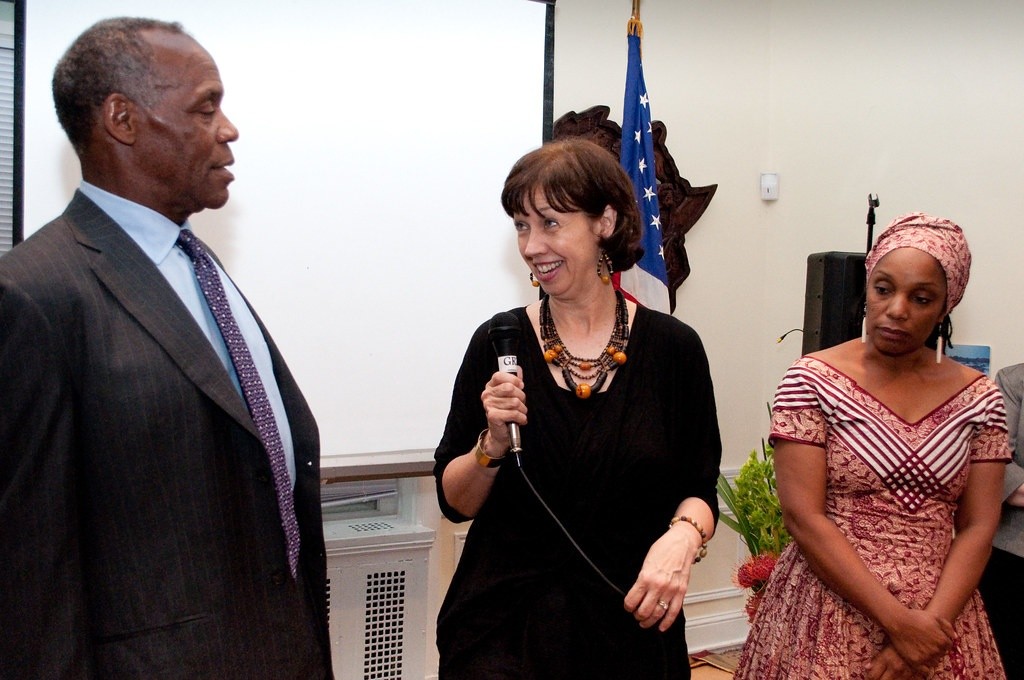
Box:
[717,443,792,623]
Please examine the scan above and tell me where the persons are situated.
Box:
[1,17,333,680]
[993,364,1024,680]
[430,139,722,680]
[736,214,1005,680]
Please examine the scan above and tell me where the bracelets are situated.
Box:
[475,429,506,467]
[670,515,708,566]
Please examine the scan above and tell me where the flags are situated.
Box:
[618,14,670,298]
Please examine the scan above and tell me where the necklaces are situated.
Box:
[535,288,633,401]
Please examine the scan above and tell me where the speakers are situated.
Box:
[802,251,868,356]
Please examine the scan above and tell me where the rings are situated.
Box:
[658,600,669,611]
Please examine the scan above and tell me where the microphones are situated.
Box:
[488,311,522,455]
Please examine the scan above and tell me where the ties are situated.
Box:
[175,228,300,582]
